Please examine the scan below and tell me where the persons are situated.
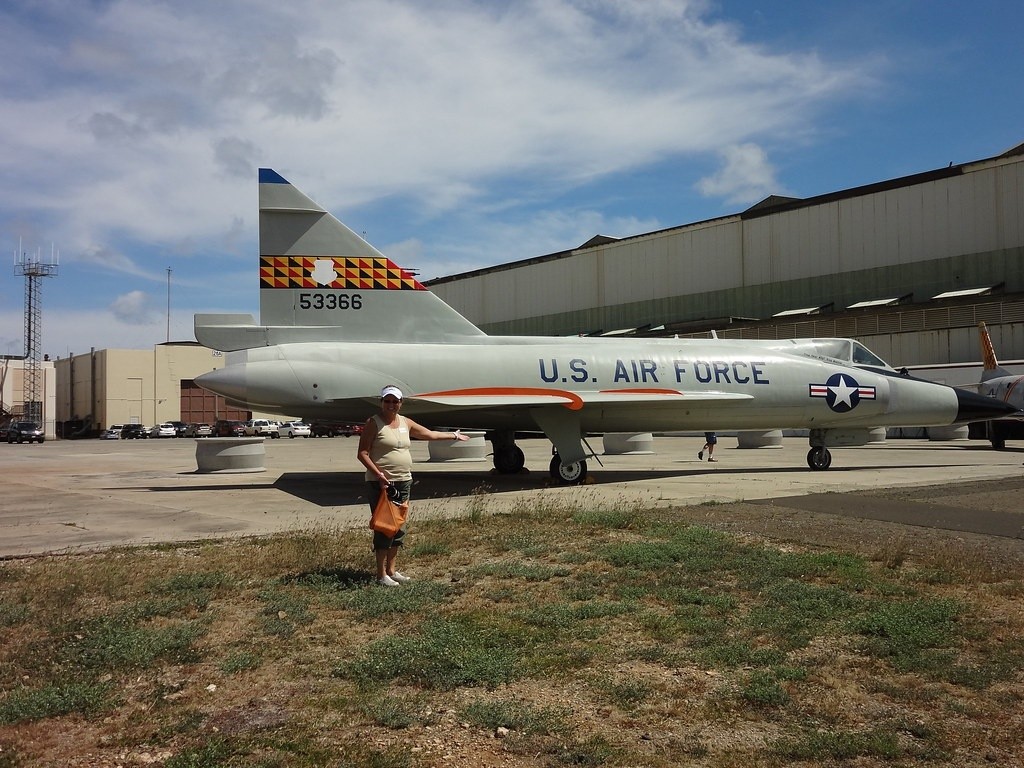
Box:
[698,432,719,463]
[357,385,471,587]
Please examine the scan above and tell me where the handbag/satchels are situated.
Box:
[372,484,410,539]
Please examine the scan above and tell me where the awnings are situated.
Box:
[845,292,914,310]
[570,302,834,337]
[931,286,994,301]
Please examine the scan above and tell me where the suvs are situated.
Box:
[309,424,353,438]
[6,420,44,443]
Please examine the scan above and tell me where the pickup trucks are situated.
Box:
[243,419,280,439]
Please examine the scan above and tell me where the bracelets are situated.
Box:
[377,472,384,481]
[453,432,458,440]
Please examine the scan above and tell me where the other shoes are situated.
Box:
[387,570,411,582]
[377,574,400,587]
[708,457,718,462]
[698,451,703,459]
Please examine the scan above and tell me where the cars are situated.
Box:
[165,420,245,437]
[149,423,176,438]
[99,423,147,440]
[274,422,311,439]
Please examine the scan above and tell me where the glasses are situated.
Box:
[382,399,402,404]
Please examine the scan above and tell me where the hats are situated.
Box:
[381,387,403,399]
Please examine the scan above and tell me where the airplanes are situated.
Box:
[193,170,1018,482]
[955,318,1024,450]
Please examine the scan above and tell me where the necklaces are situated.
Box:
[381,413,402,448]
